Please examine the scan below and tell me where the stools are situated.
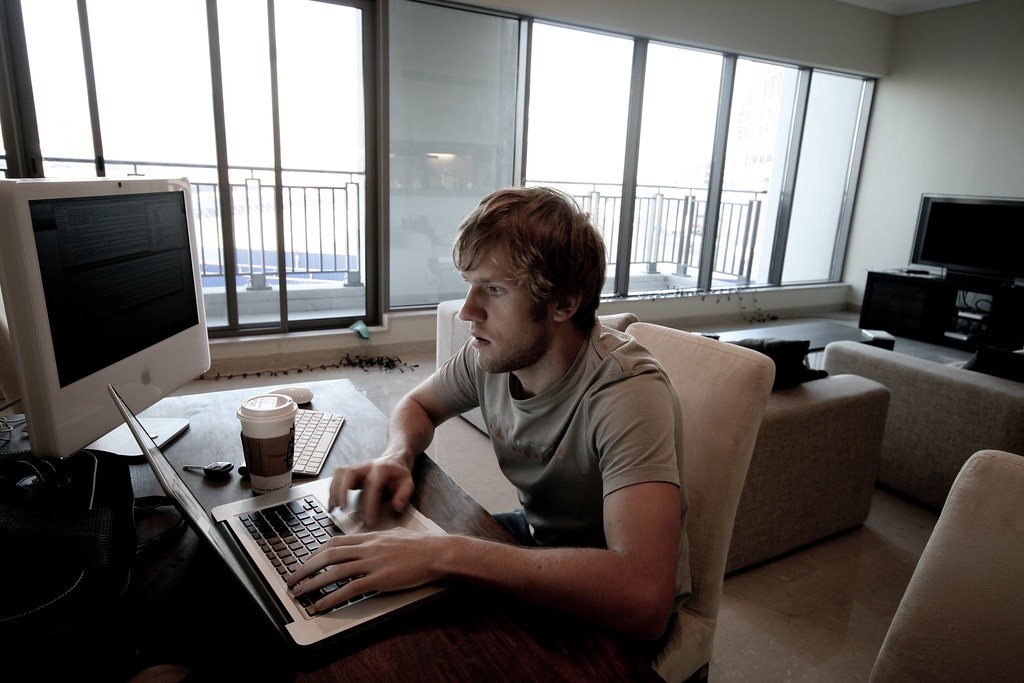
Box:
[861,329,895,352]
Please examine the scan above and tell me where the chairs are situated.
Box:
[625,322,775,683]
[868,451,1024,683]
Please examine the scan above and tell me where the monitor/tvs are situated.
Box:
[0,177,211,460]
[917,198,1024,287]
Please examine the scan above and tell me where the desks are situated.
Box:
[0,378,665,683]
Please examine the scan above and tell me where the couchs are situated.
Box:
[435,298,890,574]
[822,341,1024,510]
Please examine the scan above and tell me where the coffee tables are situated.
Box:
[691,322,873,370]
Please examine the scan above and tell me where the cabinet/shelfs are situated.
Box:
[858,270,1024,356]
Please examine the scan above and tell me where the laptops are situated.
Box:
[103,384,449,645]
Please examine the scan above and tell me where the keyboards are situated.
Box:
[238,411,343,481]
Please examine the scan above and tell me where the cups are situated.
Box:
[236,394,299,495]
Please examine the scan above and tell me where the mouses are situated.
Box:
[267,387,313,404]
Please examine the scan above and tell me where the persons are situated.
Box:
[287,186,692,666]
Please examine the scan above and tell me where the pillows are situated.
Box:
[960,346,1024,384]
[727,338,809,389]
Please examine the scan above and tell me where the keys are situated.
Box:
[183,462,234,475]
